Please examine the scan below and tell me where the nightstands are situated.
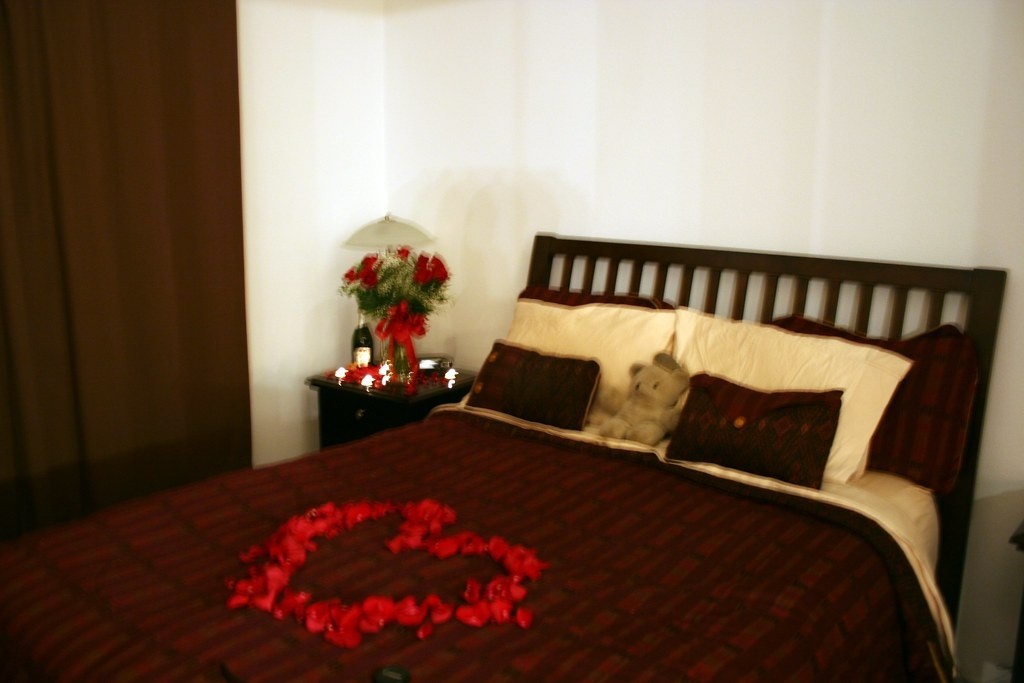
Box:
[305,365,476,450]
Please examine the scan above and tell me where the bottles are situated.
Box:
[351,311,374,366]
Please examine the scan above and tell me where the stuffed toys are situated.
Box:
[599,353,690,446]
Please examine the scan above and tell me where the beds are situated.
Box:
[1,230,1010,683]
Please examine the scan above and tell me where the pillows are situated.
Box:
[662,306,914,484]
[767,312,983,499]
[463,338,603,433]
[501,296,677,428]
[663,371,846,494]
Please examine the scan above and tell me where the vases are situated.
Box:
[390,333,415,375]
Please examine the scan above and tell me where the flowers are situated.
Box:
[339,243,454,364]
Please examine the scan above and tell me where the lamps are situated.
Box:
[345,216,443,362]
